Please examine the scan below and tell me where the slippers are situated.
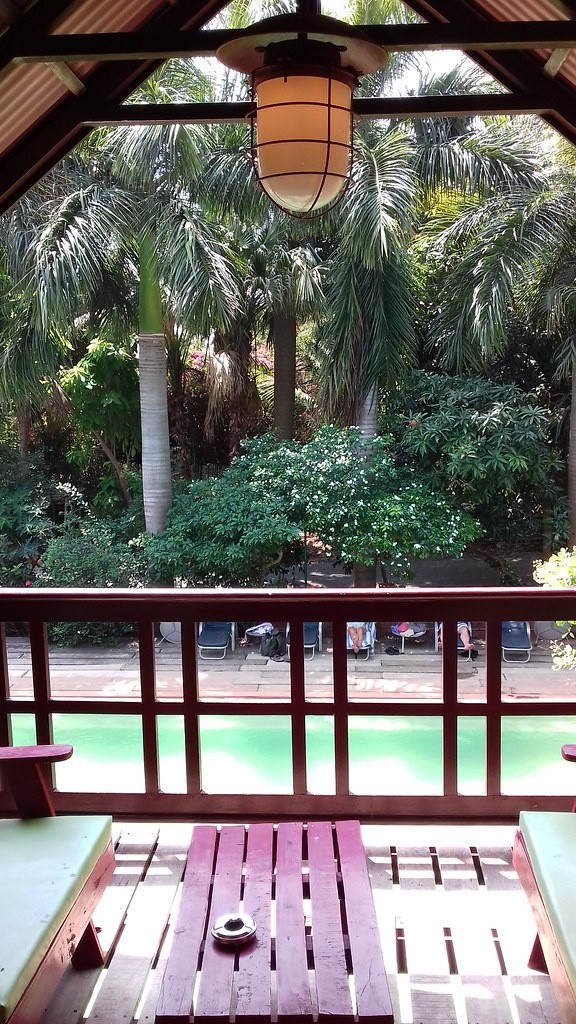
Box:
[385,647,399,655]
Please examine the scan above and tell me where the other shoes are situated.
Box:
[460,649,478,658]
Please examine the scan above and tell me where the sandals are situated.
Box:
[270,654,284,662]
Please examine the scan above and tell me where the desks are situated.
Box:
[246,628,279,639]
[391,623,428,654]
[157,622,181,647]
[155,820,394,1024]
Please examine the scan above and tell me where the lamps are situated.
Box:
[215,0,389,219]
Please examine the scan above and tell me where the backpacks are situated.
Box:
[261,629,285,656]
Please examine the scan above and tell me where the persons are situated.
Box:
[347,621,367,652]
[438,621,476,651]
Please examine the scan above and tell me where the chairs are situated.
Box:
[346,622,376,661]
[286,622,323,661]
[501,621,533,662]
[511,744,576,1024]
[0,744,117,1024]
[197,621,238,660]
[435,622,472,662]
